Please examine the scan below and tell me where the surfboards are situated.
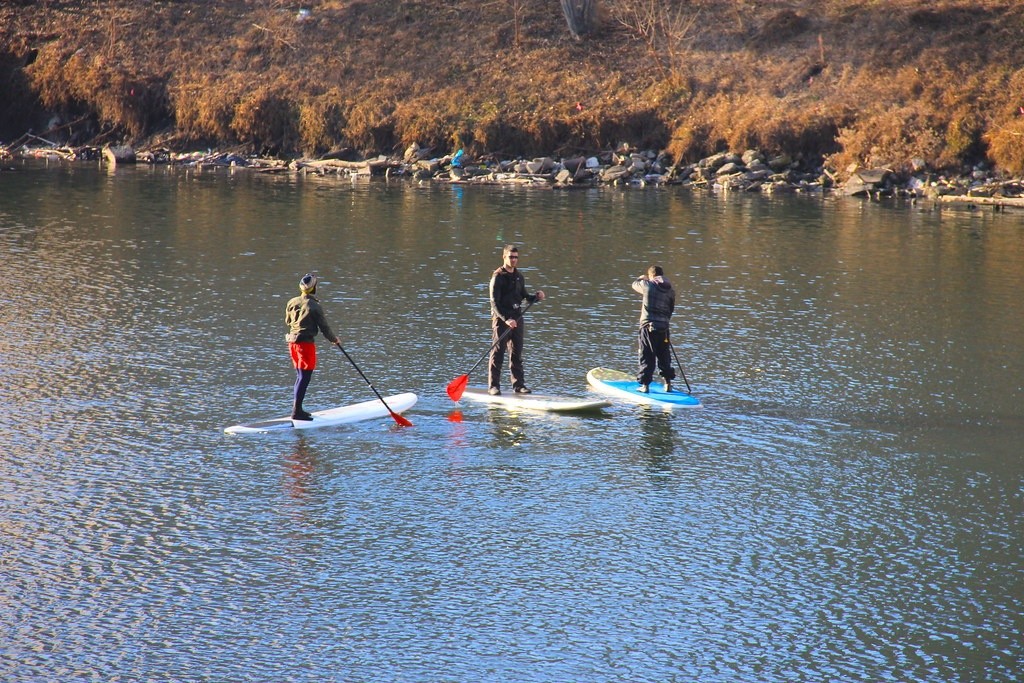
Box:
[586,366,704,410]
[223,392,418,436]
[460,388,614,413]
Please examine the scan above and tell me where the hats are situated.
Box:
[298,273,317,294]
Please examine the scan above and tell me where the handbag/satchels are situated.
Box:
[647,320,670,334]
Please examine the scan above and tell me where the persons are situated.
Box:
[576,103,583,111]
[907,189,916,205]
[633,144,639,152]
[487,244,545,395]
[450,150,463,168]
[622,142,630,156]
[631,266,676,393]
[284,273,342,421]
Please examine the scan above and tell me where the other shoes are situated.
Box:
[487,386,501,395]
[664,384,672,392]
[636,386,648,392]
[519,387,531,393]
[291,409,313,421]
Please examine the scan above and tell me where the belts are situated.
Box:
[504,303,520,310]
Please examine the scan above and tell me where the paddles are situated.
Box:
[336,344,412,427]
[669,342,692,393]
[446,299,537,402]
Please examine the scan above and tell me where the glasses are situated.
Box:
[508,255,518,259]
